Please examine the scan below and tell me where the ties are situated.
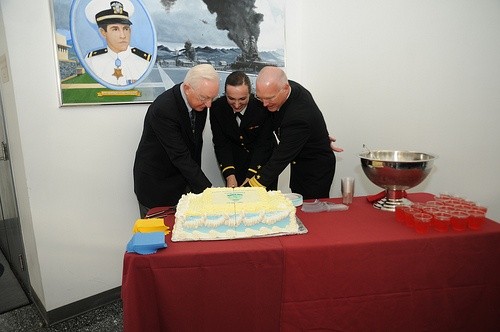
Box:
[190,110,195,133]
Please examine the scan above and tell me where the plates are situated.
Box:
[283,193,303,206]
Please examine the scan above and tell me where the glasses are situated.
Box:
[189,85,216,104]
[256,85,285,101]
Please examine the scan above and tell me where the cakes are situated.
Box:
[172,186,299,240]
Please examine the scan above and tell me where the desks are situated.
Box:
[120,194,500,332]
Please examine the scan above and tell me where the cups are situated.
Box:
[395,193,488,234]
[341,175,355,204]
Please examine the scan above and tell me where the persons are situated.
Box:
[132,64,222,219]
[242,66,344,200]
[210,71,280,192]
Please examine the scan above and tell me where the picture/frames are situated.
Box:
[48,0,287,108]
